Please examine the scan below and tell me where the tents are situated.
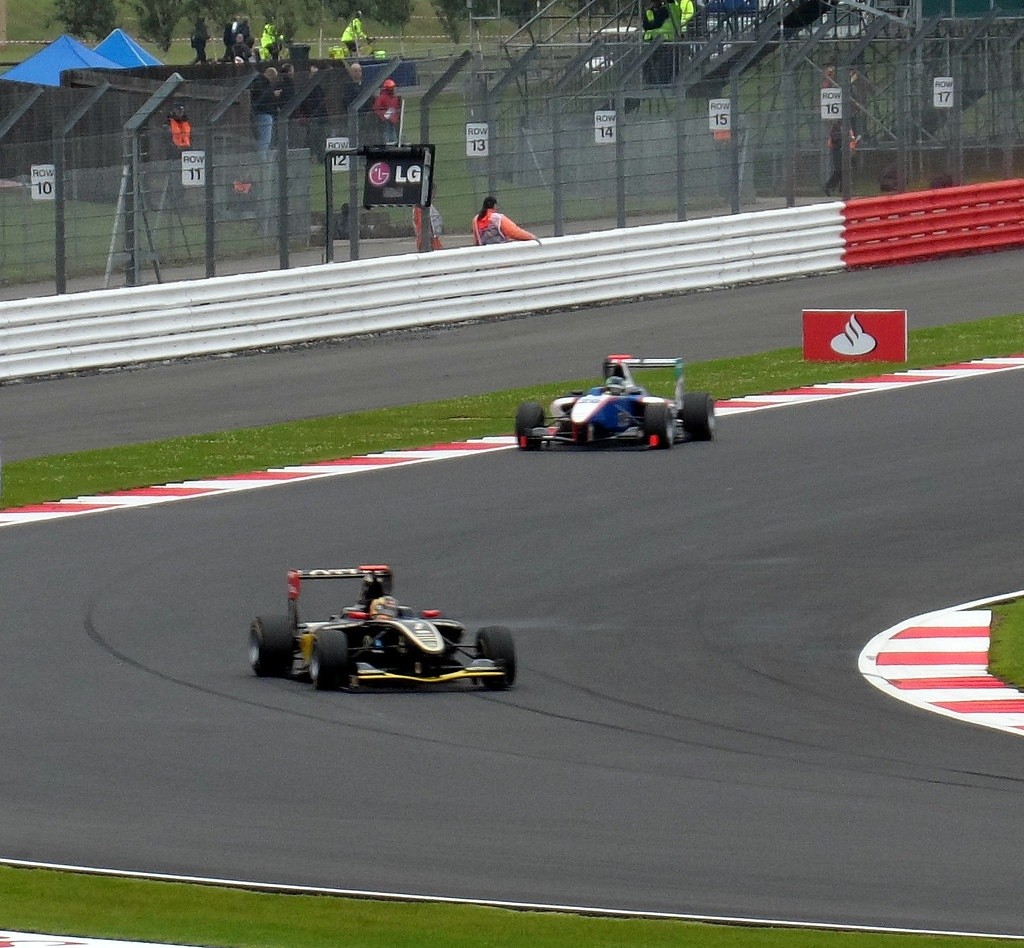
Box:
[91,29,165,68]
[0,34,126,86]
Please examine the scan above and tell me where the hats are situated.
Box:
[174,102,185,109]
[236,34,243,42]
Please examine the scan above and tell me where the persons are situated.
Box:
[369,596,399,622]
[823,120,855,198]
[472,195,543,247]
[643,0,695,85]
[601,377,629,396]
[162,11,443,252]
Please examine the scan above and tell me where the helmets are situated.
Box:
[605,377,626,389]
[370,596,398,621]
[383,79,395,87]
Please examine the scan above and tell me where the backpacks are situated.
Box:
[191,30,202,48]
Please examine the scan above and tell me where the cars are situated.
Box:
[797,8,870,38]
[582,27,638,75]
[688,0,776,67]
